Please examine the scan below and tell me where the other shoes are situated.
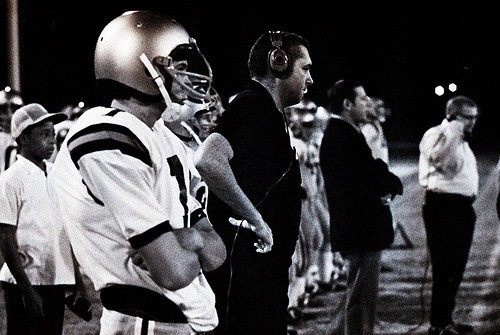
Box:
[287,269,345,334]
[426,322,473,335]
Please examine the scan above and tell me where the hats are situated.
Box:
[11,103,67,140]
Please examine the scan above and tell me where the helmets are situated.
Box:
[94,11,191,101]
[162,84,216,137]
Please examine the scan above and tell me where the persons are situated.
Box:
[0,11,403,335]
[418,97,478,335]
